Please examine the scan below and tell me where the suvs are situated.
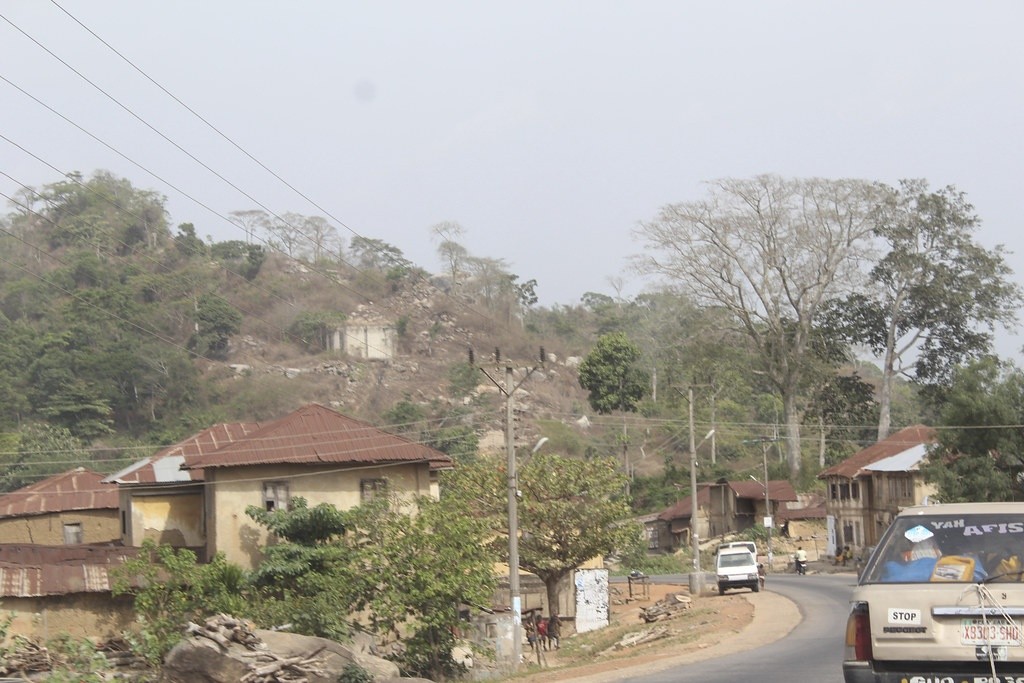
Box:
[842,501,1024,683]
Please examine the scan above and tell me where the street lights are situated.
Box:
[690,429,716,595]
[507,435,549,668]
[750,475,774,572]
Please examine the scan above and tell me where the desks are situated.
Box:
[627,574,651,601]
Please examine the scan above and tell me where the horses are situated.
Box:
[547,615,563,650]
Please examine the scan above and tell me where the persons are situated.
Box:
[758,564,768,590]
[794,547,807,571]
[524,614,562,652]
[832,546,853,567]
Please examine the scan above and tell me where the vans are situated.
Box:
[715,548,762,597]
[718,541,756,565]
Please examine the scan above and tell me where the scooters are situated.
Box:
[794,557,806,575]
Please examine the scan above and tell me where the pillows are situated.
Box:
[900,537,942,564]
[880,554,988,582]
